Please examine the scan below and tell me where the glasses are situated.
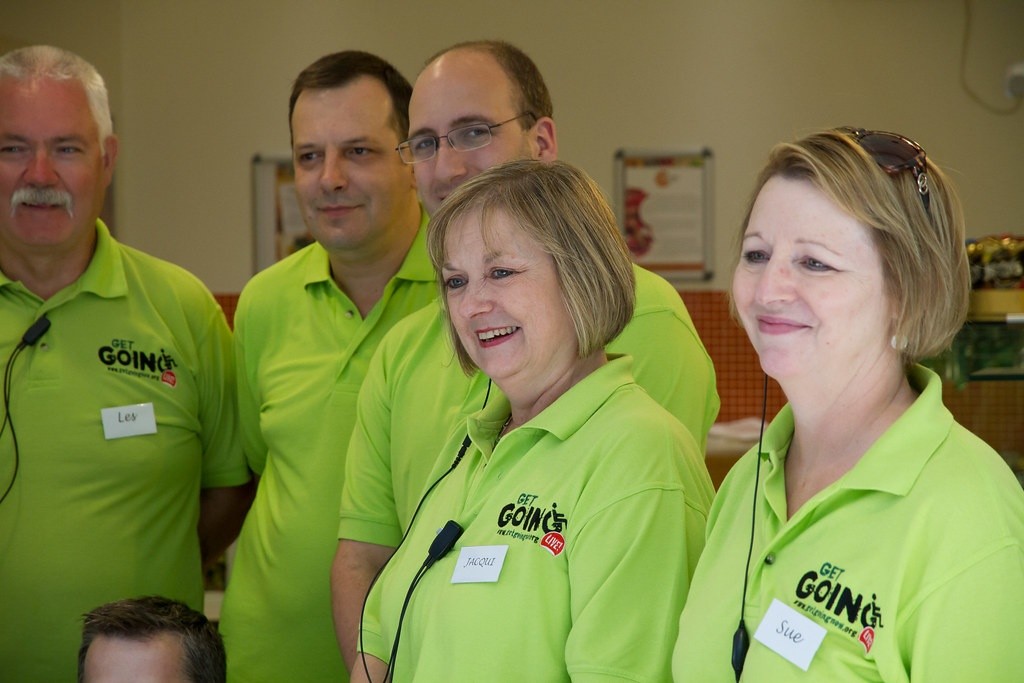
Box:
[394,111,538,164]
[834,126,932,219]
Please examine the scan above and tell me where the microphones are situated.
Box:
[424,520,465,568]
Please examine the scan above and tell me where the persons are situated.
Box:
[331,41,720,675]
[350,159,716,683]
[670,127,1024,683]
[218,51,442,683]
[0,46,251,683]
[78,596,226,683]
